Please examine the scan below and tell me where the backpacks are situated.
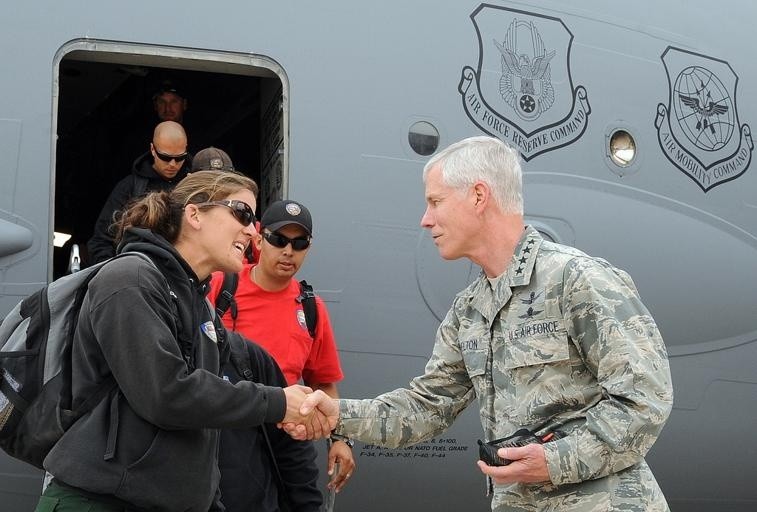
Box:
[0,251,188,472]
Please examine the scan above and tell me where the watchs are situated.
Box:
[332,436,355,448]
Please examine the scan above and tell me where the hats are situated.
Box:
[153,77,180,97]
[191,146,235,171]
[261,199,314,241]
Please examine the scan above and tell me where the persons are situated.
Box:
[277,134,674,512]
[208,329,323,512]
[191,146,261,265]
[86,121,194,267]
[152,78,187,125]
[33,169,330,512]
[207,200,356,512]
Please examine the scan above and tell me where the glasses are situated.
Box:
[262,231,311,252]
[189,200,258,228]
[152,141,189,163]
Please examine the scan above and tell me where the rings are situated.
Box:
[347,474,352,479]
